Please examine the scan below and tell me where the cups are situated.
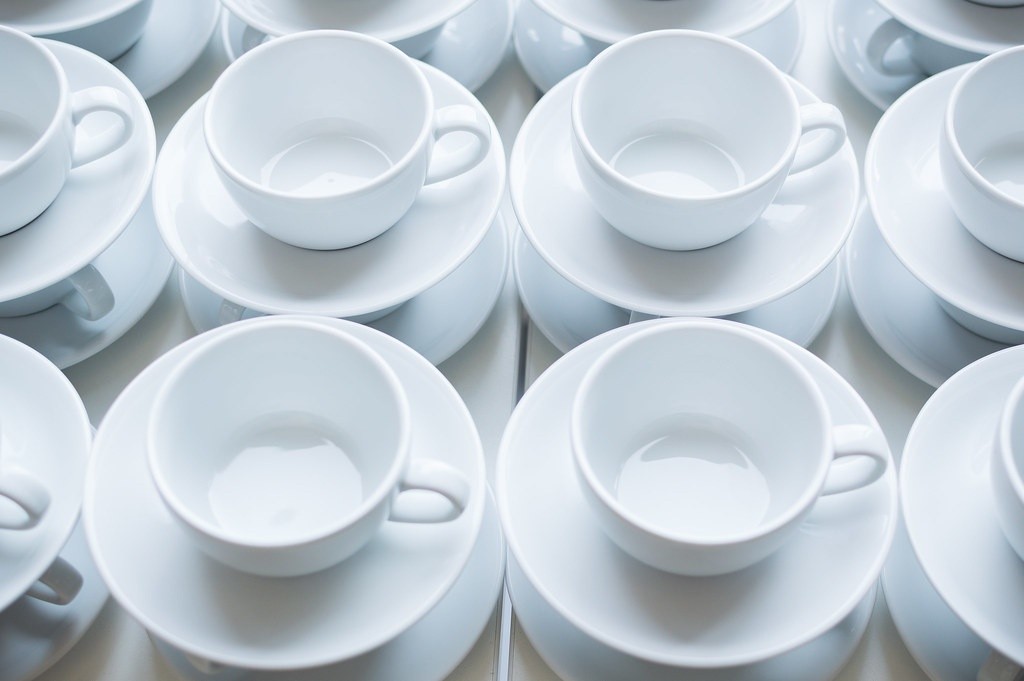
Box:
[0,23,133,238]
[991,377,1024,562]
[203,28,493,251]
[938,45,1024,264]
[567,320,888,578]
[568,29,847,251]
[141,322,470,577]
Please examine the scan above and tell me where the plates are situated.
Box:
[0,0,1024,681]
